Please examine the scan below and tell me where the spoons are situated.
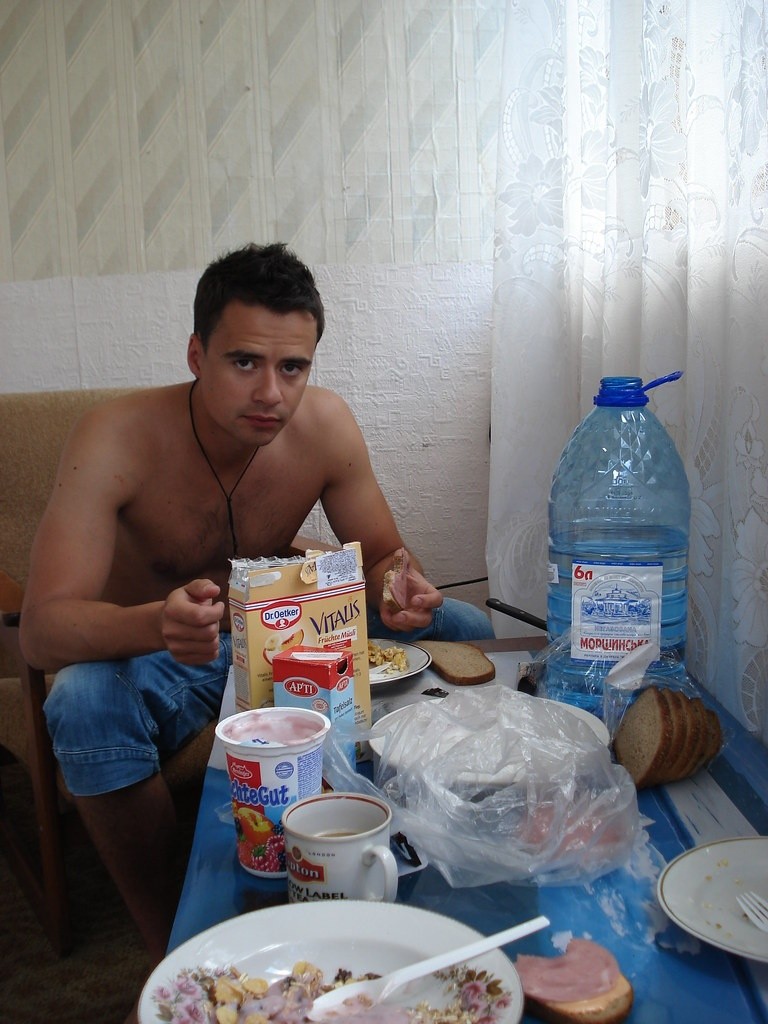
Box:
[304,915,550,1024]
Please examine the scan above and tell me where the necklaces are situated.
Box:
[189,377,261,557]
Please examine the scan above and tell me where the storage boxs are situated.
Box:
[272,645,356,772]
[226,541,372,762]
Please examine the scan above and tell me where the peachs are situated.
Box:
[279,628,304,652]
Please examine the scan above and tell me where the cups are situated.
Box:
[281,791,399,903]
[215,706,331,880]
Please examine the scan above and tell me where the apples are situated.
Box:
[236,807,273,845]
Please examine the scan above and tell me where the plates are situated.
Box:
[137,899,524,1024]
[657,836,768,964]
[369,694,611,786]
[366,639,432,686]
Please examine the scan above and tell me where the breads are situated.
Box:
[614,686,723,789]
[410,640,496,686]
[515,937,633,1024]
[382,547,409,613]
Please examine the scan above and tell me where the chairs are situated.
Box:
[0,388,151,956]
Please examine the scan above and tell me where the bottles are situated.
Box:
[546,368,691,723]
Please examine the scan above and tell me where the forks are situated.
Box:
[736,890,768,932]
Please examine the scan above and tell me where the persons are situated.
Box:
[19,240,499,1024]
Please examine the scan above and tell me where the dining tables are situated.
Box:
[165,636,768,1024]
